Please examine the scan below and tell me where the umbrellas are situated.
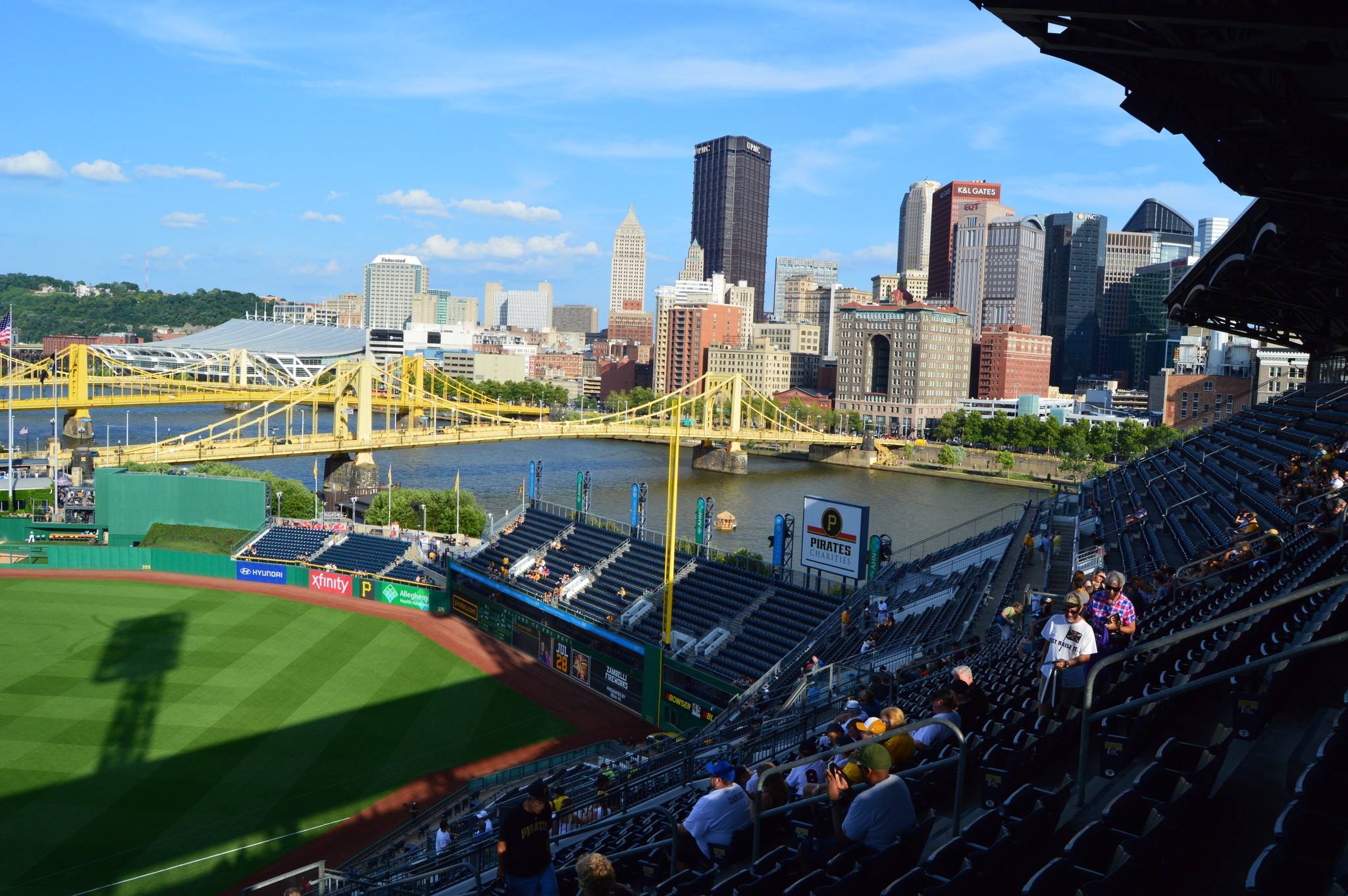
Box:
[180,467,190,470]
[52,476,73,486]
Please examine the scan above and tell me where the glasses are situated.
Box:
[1105,582,1122,592]
[1242,514,1246,515]
[1062,606,1081,614]
[1344,476,1348,477]
[1034,613,1041,617]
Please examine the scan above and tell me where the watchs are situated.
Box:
[1066,661,1069,668]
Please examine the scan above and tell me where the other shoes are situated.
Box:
[1027,563,1034,566]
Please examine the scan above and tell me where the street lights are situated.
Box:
[202,386,206,400]
[50,418,55,435]
[36,437,39,451]
[248,385,251,399]
[127,411,130,446]
[199,435,202,458]
[95,386,99,396]
[12,416,15,453]
[168,428,171,440]
[57,390,59,406]
[420,504,427,532]
[158,388,161,402]
[110,388,114,404]
[118,440,121,465]
[271,382,929,453]
[78,426,85,446]
[487,512,493,535]
[91,432,94,448]
[349,496,359,524]
[229,383,233,391]
[1,392,6,408]
[154,417,158,462]
[42,388,46,398]
[266,405,270,438]
[269,383,272,391]
[274,491,284,518]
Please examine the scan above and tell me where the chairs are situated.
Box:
[234,382,1348,896]
[11,491,98,540]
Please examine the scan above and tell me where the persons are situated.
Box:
[8,439,233,543]
[996,411,1348,721]
[284,665,988,896]
[245,518,469,590]
[539,640,547,664]
[730,599,981,689]
[683,428,973,466]
[485,515,666,670]
[253,423,658,448]
[544,645,551,666]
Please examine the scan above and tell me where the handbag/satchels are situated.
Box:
[996,611,1010,626]
[1039,538,1044,552]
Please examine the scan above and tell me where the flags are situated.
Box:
[19,425,28,434]
[455,472,458,492]
[388,467,392,485]
[313,460,317,480]
[38,352,55,385]
[0,311,10,345]
[518,481,523,500]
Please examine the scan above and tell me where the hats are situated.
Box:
[593,775,607,785]
[1208,547,1216,553]
[821,700,892,771]
[551,786,565,795]
[706,761,735,781]
[529,782,551,801]
[1033,588,1038,592]
[1032,611,1046,619]
[1125,581,1138,591]
[950,679,969,694]
[1046,598,1052,603]
[847,673,855,677]
[1029,531,1034,532]
[1344,471,1348,474]
[1152,574,1164,583]
[927,688,951,701]
[1290,460,1298,464]
[1240,510,1278,535]
[476,811,488,818]
[1061,592,1083,607]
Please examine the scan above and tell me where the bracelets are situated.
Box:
[1116,626,1120,632]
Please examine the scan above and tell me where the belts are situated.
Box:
[1054,546,1061,547]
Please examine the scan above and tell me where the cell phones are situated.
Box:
[828,761,836,782]
